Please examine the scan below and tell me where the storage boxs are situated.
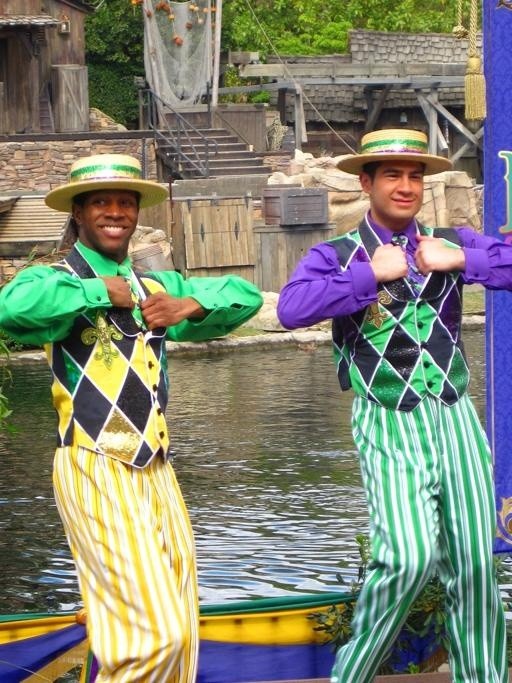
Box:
[264,184,330,226]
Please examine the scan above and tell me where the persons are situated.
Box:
[274,126,511,682]
[0,151,265,682]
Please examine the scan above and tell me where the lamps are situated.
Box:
[55,12,71,34]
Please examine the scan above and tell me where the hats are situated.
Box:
[45,155,171,213]
[336,128,454,180]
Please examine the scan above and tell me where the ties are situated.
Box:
[117,266,151,333]
[391,235,427,296]
